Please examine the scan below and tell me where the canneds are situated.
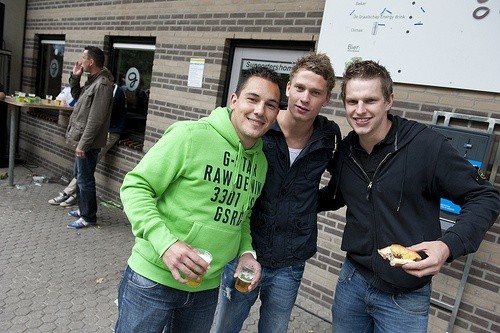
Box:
[59,174,71,186]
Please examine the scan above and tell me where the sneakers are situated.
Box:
[60,195,77,206]
[68,209,80,217]
[67,217,96,229]
[48,193,68,204]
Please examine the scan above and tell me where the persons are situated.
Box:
[115,67,281,333]
[314,61,500,332]
[0,84,6,101]
[49,46,129,230]
[213,54,342,333]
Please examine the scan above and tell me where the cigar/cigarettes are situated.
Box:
[80,65,83,67]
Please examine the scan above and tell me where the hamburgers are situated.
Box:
[377,245,421,266]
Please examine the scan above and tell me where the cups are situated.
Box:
[29,93,36,104]
[184,248,214,289]
[234,264,256,293]
[19,92,26,102]
[15,91,20,102]
[55,97,62,106]
[46,94,53,104]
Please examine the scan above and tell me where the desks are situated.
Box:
[0,96,76,189]
